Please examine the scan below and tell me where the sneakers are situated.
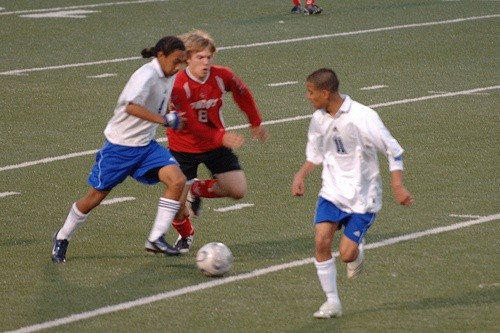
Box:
[304,5,322,15]
[347,244,365,280]
[185,178,200,218]
[173,234,194,253]
[51,230,69,263]
[144,233,180,256]
[313,301,342,318]
[291,6,302,13]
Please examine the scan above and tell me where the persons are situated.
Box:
[291,67,415,319]
[291,0,321,16]
[51,36,188,264]
[165,29,268,254]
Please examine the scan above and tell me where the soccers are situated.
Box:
[196,242,234,277]
